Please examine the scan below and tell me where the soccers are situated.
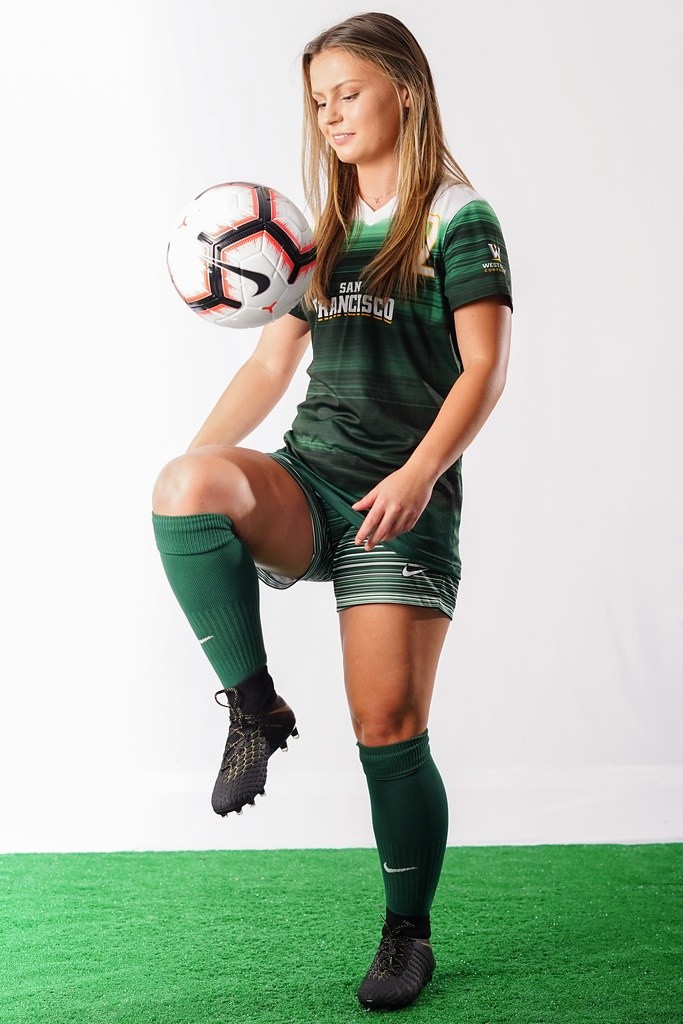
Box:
[164,180,317,328]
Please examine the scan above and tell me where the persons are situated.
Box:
[151,12,512,1014]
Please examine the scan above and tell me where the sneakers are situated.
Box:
[211,696,300,817]
[357,932,437,1011]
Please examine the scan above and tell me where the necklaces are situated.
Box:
[360,188,395,204]
